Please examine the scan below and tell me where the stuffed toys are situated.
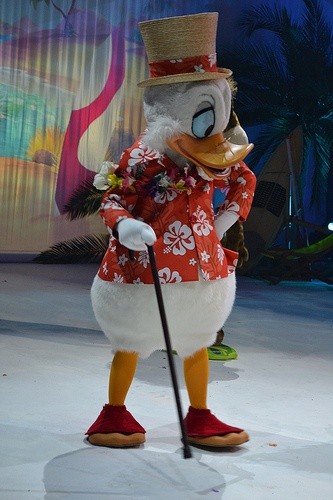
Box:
[83,14,256,450]
